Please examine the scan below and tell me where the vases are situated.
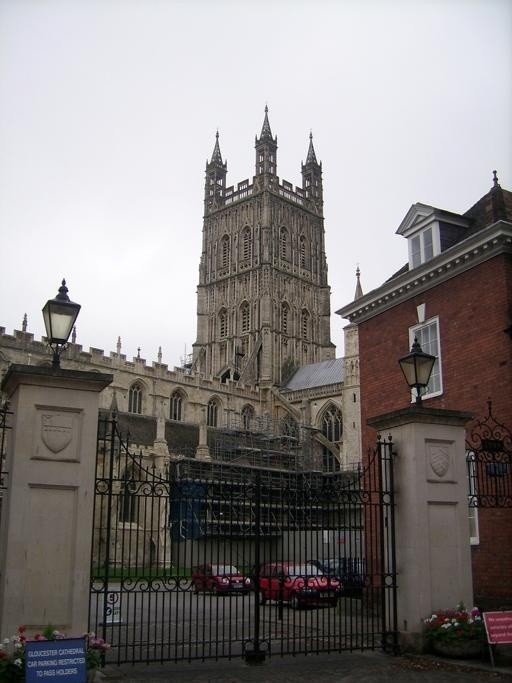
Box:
[432,639,486,658]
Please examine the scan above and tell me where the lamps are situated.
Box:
[42,278,81,369]
[398,336,436,410]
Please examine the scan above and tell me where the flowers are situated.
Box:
[421,600,488,648]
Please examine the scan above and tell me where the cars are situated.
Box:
[320,549,367,601]
[191,559,251,594]
[253,559,341,609]
